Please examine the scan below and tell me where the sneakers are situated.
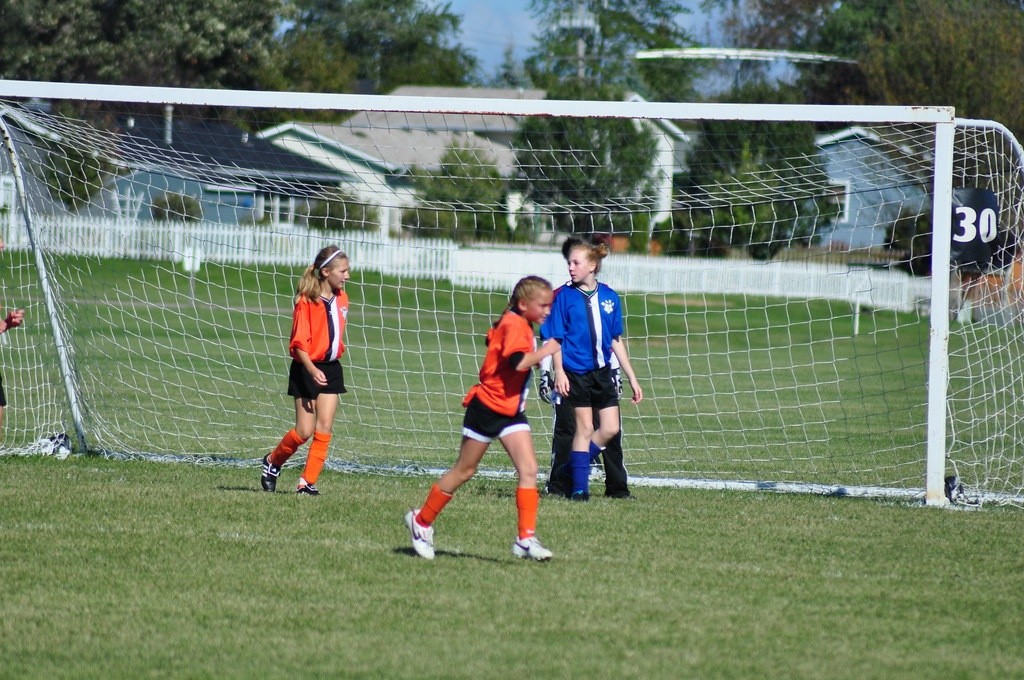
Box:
[295,483,320,496]
[404,508,435,560]
[261,453,281,492]
[512,536,553,561]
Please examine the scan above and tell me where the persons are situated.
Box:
[261,246,351,494]
[0,239,25,426]
[538,234,643,502]
[956,173,1000,324]
[404,276,561,561]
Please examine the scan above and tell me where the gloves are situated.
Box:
[611,367,623,401]
[539,370,554,403]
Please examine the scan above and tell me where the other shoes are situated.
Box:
[619,494,637,501]
[555,464,571,499]
[572,492,589,503]
